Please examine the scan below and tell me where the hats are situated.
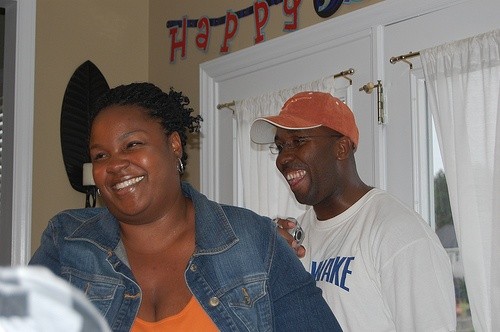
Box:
[250,90,359,146]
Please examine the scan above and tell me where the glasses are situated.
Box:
[268,135,341,155]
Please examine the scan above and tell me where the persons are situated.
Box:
[247,90,459,332]
[27,80,342,332]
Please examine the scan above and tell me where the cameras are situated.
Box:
[284,217,305,246]
